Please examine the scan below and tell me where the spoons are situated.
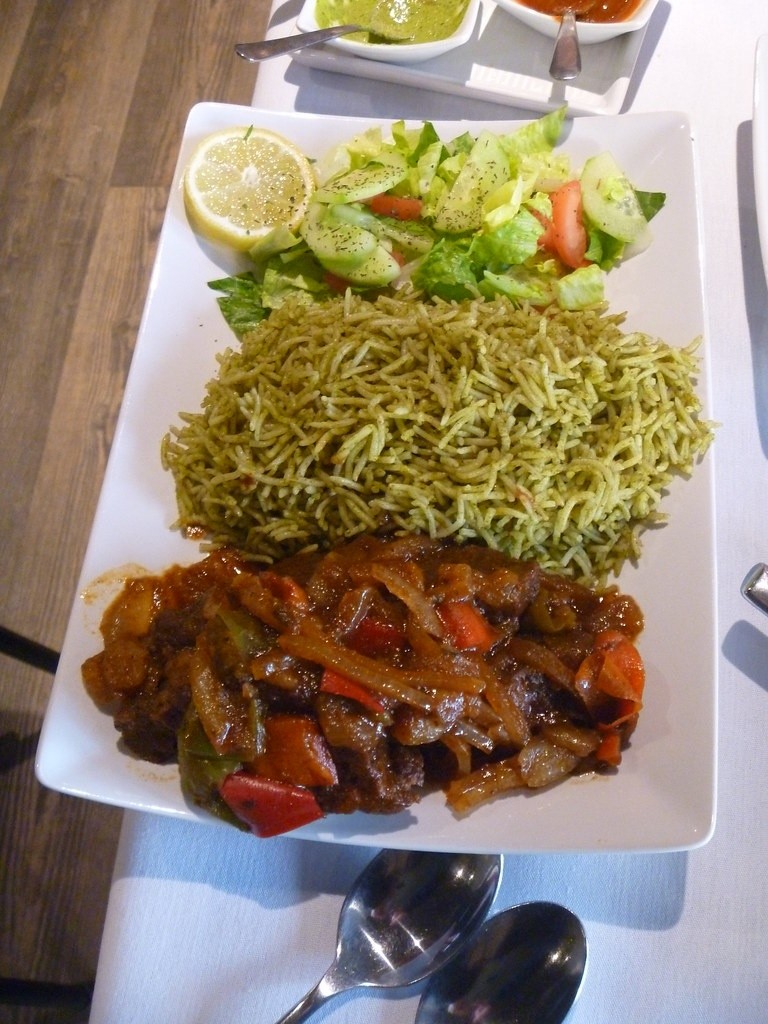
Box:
[548,3,595,80]
[276,849,503,1024]
[236,0,408,63]
[414,902,587,1024]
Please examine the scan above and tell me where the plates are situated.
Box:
[35,100,716,854]
[496,0,660,46]
[291,20,651,118]
[295,0,481,65]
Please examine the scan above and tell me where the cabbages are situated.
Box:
[208,101,667,332]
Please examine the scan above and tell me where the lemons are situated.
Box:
[183,127,316,248]
